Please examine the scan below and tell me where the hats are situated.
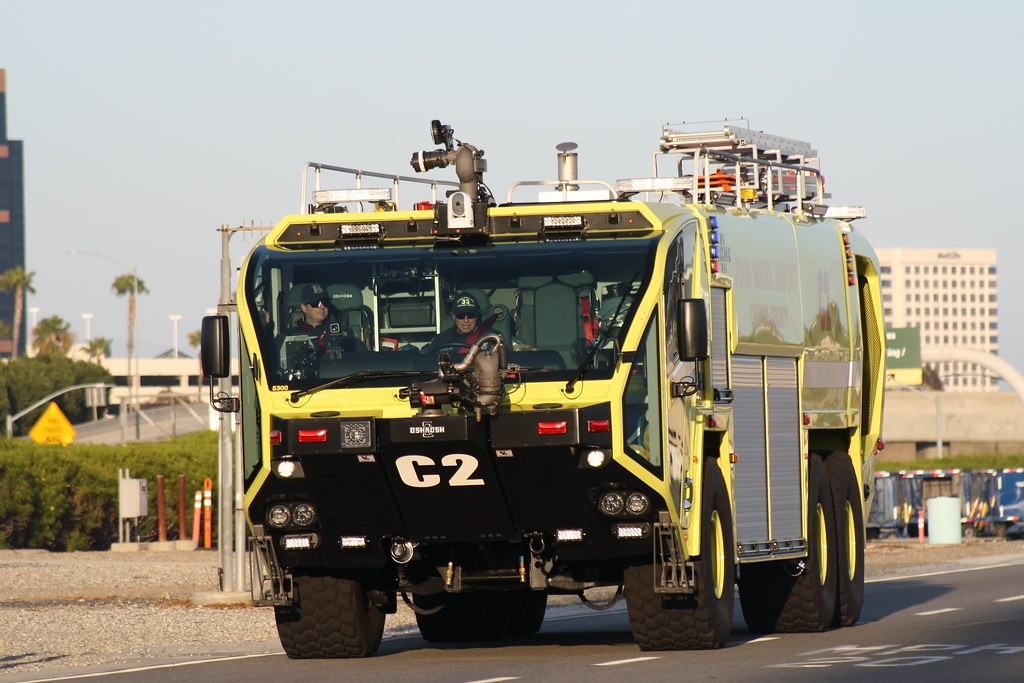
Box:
[450,293,481,315]
[301,284,333,304]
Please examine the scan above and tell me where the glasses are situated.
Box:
[454,313,476,319]
[308,299,331,308]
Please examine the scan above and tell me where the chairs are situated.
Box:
[285,282,373,352]
[441,287,511,351]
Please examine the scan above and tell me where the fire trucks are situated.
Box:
[200,118,884,659]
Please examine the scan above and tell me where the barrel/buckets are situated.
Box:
[927,497,962,546]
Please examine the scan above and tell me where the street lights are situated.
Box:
[29,307,39,328]
[82,313,94,341]
[169,315,181,360]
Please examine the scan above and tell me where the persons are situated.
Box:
[430,292,510,353]
[273,284,363,352]
